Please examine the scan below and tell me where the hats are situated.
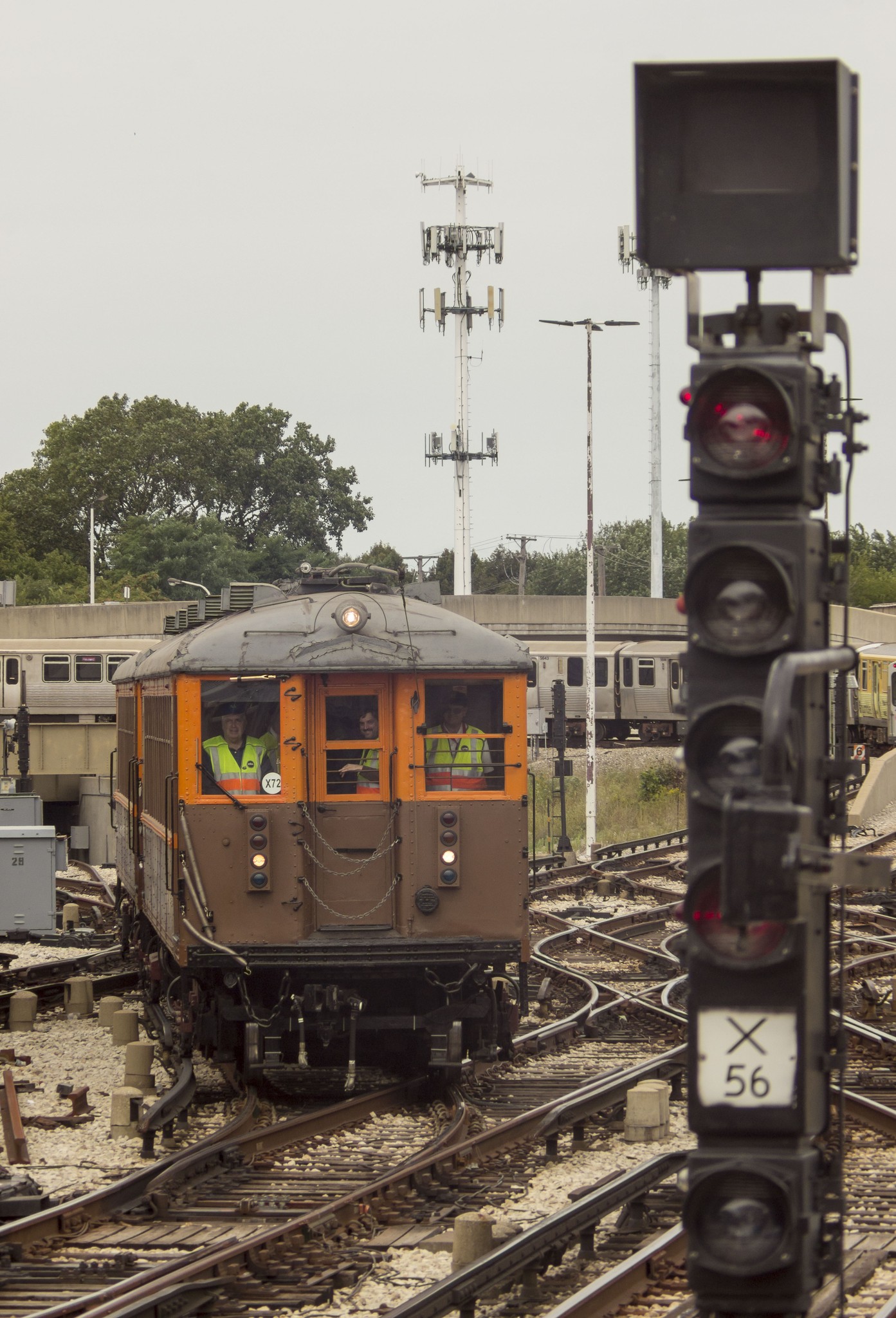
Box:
[448,691,467,706]
[222,702,245,714]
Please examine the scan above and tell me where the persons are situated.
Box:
[202,702,275,794]
[425,691,494,791]
[339,708,379,794]
[259,704,280,774]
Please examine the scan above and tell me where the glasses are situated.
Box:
[446,708,462,714]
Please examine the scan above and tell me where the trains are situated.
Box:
[0,646,896,756]
[108,562,530,1090]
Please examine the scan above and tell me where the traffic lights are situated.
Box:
[634,58,865,281]
[674,348,831,1308]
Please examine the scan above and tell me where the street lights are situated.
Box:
[89,494,108,604]
[539,315,640,846]
